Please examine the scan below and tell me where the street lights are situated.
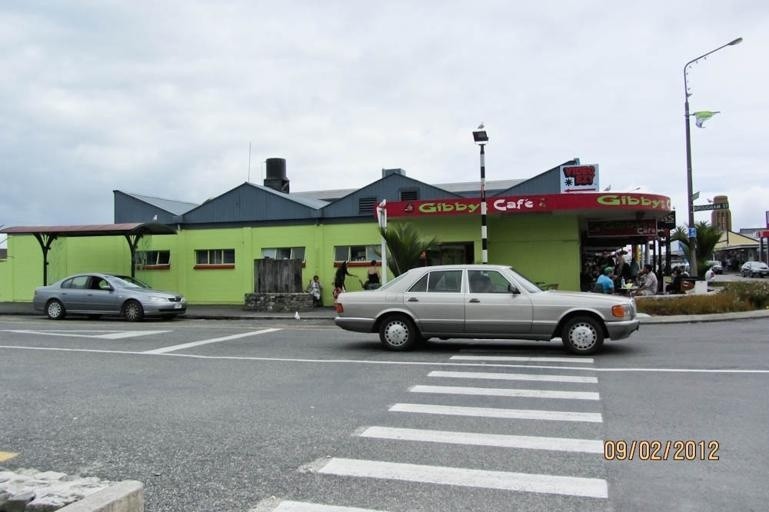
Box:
[472,124,488,277]
[684,38,743,277]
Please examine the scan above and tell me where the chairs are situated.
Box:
[590,283,603,292]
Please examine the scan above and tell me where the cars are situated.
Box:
[741,261,769,278]
[334,264,639,355]
[707,261,722,274]
[34,273,186,322]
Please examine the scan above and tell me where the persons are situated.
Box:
[334,259,359,293]
[704,264,715,282]
[367,259,380,290]
[309,275,323,307]
[583,254,691,295]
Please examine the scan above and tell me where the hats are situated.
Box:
[603,266,616,274]
